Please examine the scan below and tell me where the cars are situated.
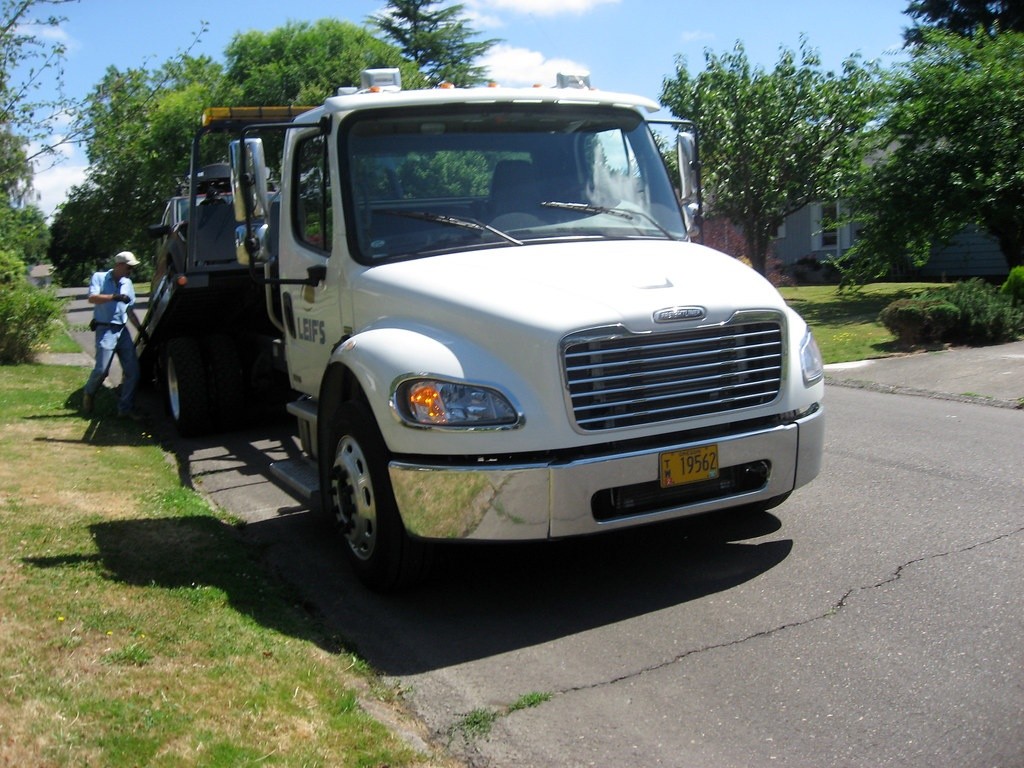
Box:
[145,192,282,293]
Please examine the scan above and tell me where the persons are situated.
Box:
[81,251,149,420]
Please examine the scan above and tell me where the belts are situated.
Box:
[96,322,114,326]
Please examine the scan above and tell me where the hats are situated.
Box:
[116,251,141,266]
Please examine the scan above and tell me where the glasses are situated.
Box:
[128,265,134,270]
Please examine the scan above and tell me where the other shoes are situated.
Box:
[117,409,143,420]
[83,393,94,411]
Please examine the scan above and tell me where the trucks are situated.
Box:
[130,64,824,591]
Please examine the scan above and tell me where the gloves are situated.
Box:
[113,293,131,304]
[138,326,149,340]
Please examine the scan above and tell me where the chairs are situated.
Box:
[493,159,542,217]
